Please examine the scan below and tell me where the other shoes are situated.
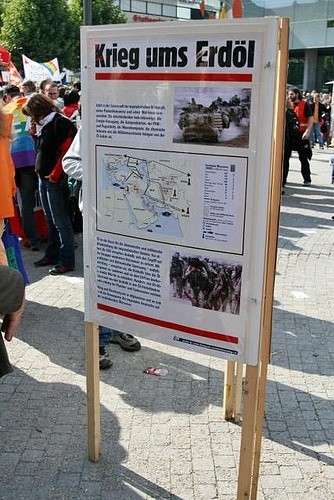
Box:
[46,262,74,276]
[34,258,56,266]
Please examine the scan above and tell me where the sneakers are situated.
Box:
[111,331,141,351]
[97,347,116,368]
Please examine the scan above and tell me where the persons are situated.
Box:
[281,87,334,220]
[169,252,242,315]
[0,79,141,380]
[185,94,250,113]
